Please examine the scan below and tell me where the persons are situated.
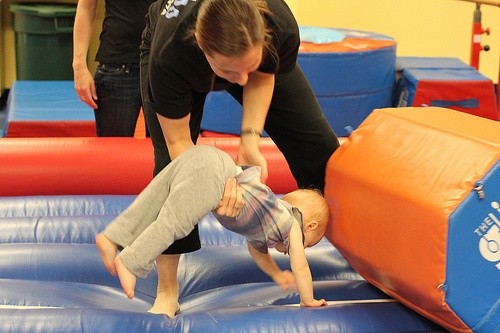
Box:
[138,1,341,319]
[72,0,157,138]
[96,145,329,309]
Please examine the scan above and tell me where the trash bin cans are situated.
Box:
[10,5,76,81]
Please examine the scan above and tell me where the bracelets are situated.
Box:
[239,128,263,139]
